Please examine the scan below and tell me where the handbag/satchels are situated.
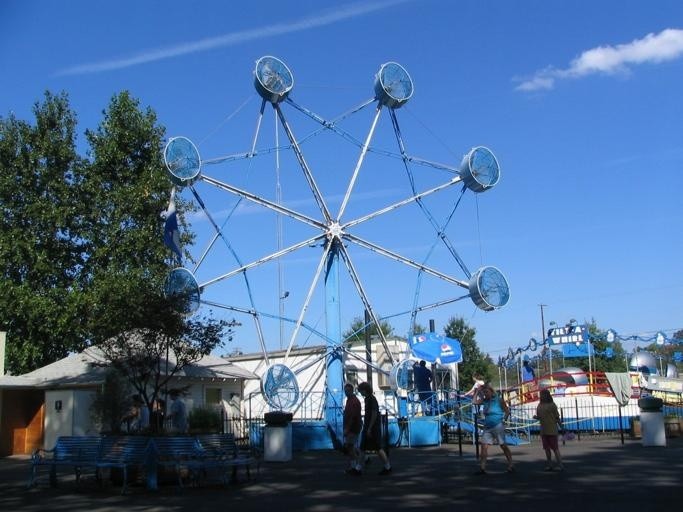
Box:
[343,443,354,457]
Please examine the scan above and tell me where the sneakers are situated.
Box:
[505,464,514,472]
[346,465,364,477]
[376,466,392,475]
[474,468,486,474]
[544,461,565,472]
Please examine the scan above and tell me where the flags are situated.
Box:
[161,187,181,261]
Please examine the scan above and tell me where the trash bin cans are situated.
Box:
[638,397,667,448]
[264,412,293,463]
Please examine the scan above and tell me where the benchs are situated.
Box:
[25,432,261,496]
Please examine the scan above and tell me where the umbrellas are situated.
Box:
[408,331,463,366]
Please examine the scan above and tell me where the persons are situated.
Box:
[343,384,362,476]
[413,360,433,414]
[519,362,535,381]
[358,382,393,475]
[167,390,188,434]
[472,385,513,475]
[533,390,564,473]
